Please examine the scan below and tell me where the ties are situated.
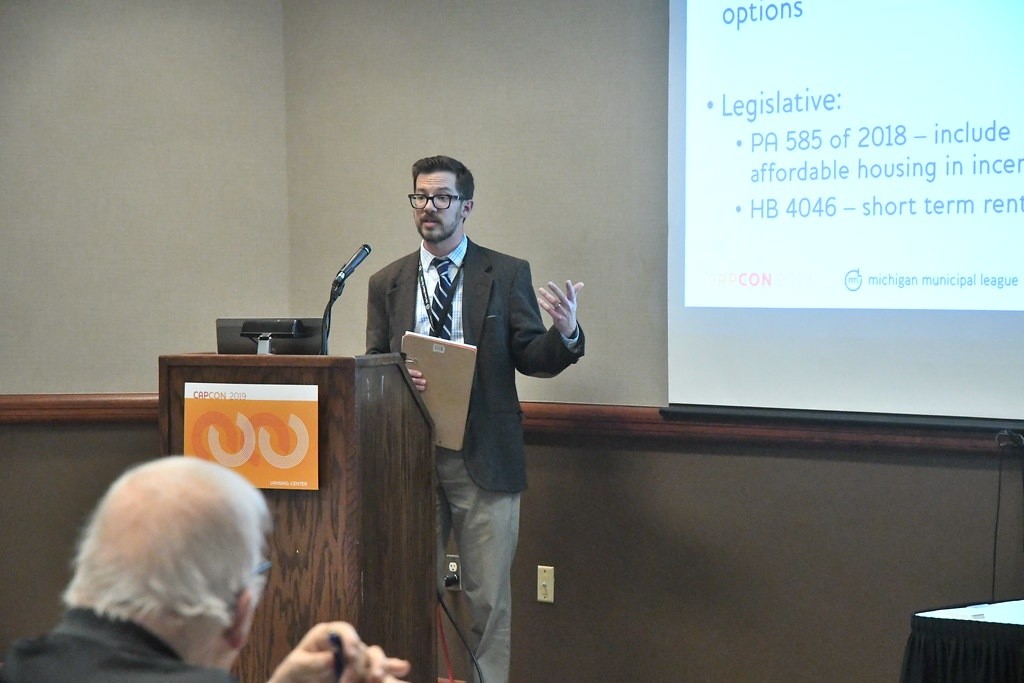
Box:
[428,258,455,341]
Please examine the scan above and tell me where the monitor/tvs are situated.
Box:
[215,319,329,356]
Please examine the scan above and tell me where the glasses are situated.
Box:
[408,192,466,211]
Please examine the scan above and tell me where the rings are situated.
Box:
[555,304,560,309]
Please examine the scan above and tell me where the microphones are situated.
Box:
[334,244,371,283]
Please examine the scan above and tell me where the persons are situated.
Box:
[366,154,585,683]
[0,456,409,683]
